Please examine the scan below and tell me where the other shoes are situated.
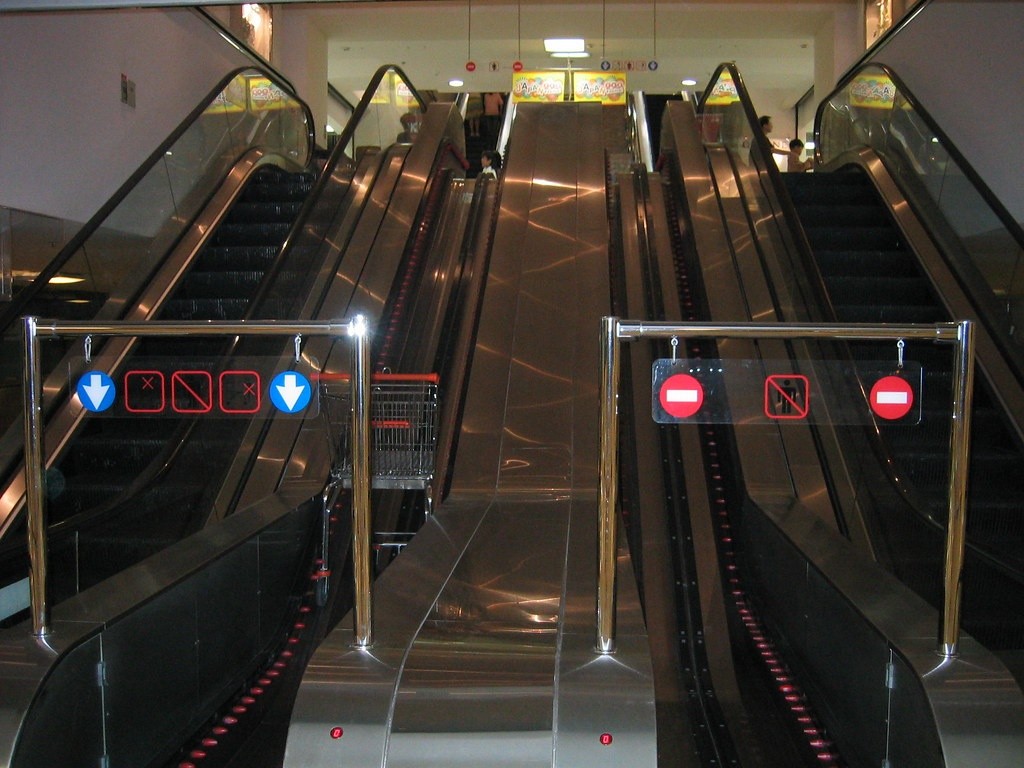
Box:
[471,133,475,137]
[476,133,480,137]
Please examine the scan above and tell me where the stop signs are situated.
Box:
[659,373,703,418]
[870,376,914,420]
[512,61,522,72]
[466,61,475,71]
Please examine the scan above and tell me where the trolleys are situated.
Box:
[309,366,441,606]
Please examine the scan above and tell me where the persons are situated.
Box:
[481,92,505,135]
[749,116,797,171]
[470,118,481,144]
[481,152,497,180]
[788,139,813,172]
